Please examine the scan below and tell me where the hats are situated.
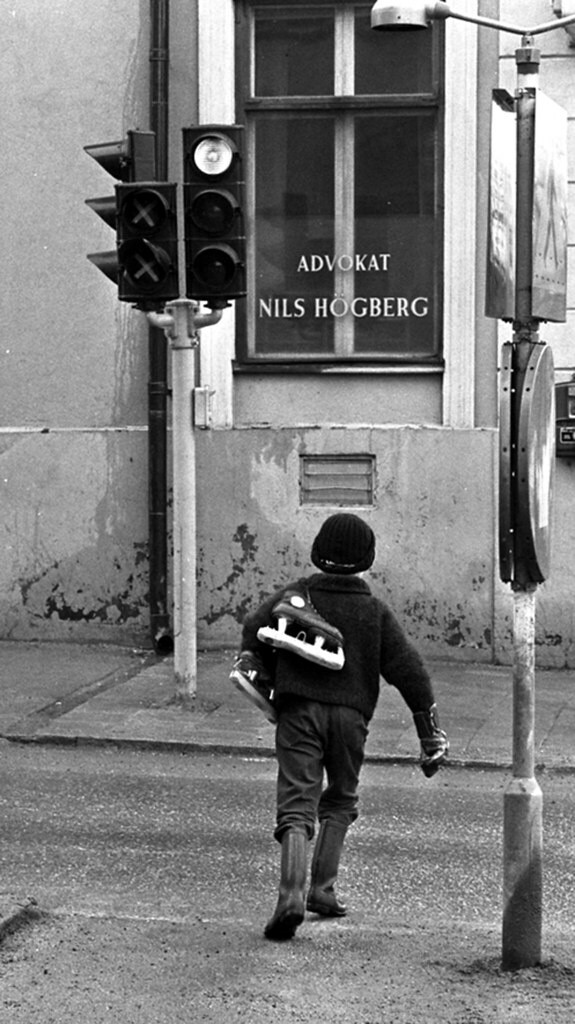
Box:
[310,513,375,575]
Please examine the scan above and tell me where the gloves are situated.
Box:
[413,705,447,778]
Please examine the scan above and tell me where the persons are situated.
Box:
[238,513,450,942]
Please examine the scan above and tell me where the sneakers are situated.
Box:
[226,651,277,721]
[256,589,345,670]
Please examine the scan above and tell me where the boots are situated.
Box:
[264,828,307,941]
[305,819,348,917]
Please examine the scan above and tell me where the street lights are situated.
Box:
[369,0,575,975]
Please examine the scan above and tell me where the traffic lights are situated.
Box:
[182,125,249,302]
[114,180,179,300]
[82,128,159,307]
